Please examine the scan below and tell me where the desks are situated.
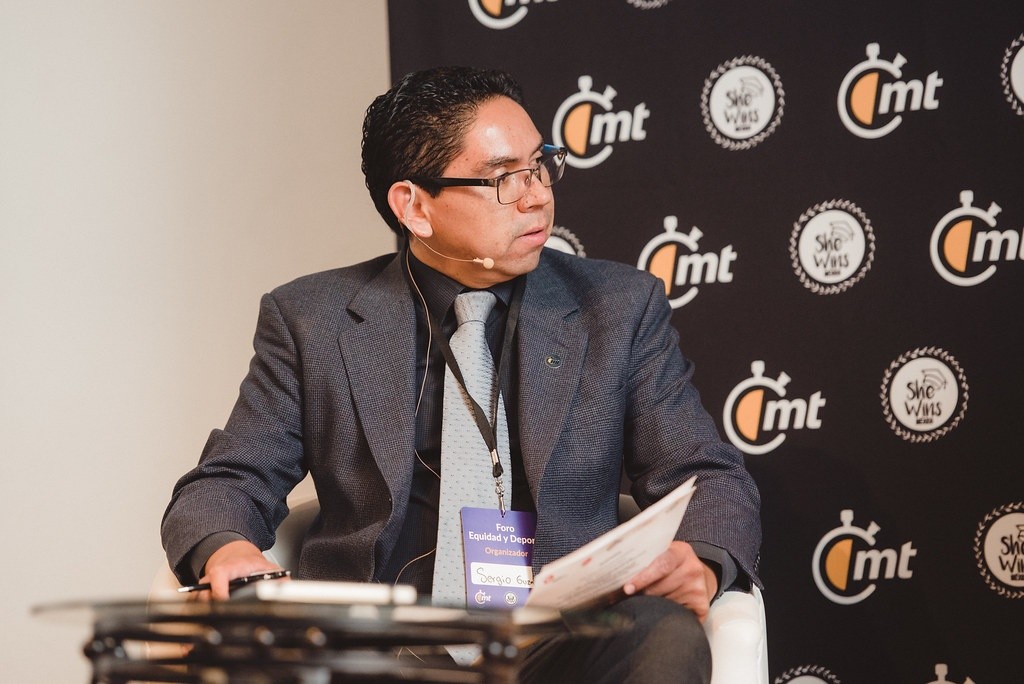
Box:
[29,599,639,682]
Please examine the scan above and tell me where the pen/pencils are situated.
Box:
[177,570,291,593]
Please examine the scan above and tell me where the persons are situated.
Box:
[159,62,764,684]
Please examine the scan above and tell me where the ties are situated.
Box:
[431,291,512,667]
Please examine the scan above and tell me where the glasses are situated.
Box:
[418,147,568,205]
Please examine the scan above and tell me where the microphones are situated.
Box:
[406,223,496,270]
